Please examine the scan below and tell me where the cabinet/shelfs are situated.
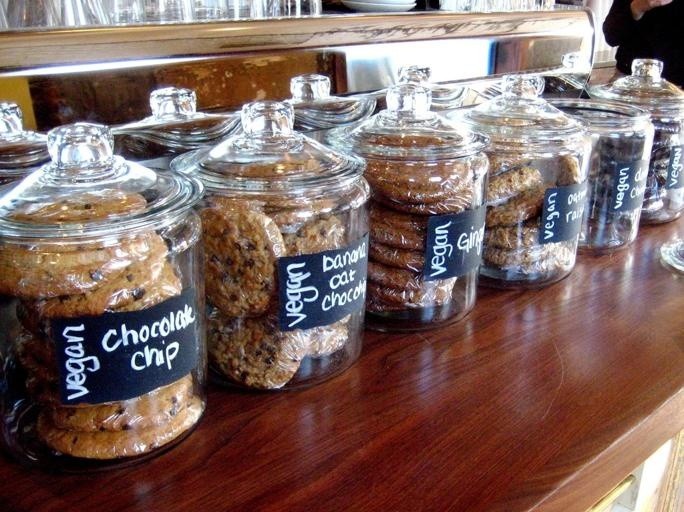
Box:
[0,5,684,512]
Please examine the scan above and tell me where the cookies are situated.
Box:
[468,106,585,280]
[0,189,205,459]
[200,148,353,392]
[353,130,486,317]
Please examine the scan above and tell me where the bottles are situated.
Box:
[406,51,470,105]
[188,99,373,392]
[357,79,485,332]
[471,69,588,296]
[0,97,60,166]
[270,64,380,121]
[618,56,683,228]
[113,81,242,144]
[566,89,650,270]
[0,114,207,469]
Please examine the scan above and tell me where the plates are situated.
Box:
[342,0,417,12]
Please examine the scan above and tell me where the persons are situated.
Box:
[601,0,682,91]
